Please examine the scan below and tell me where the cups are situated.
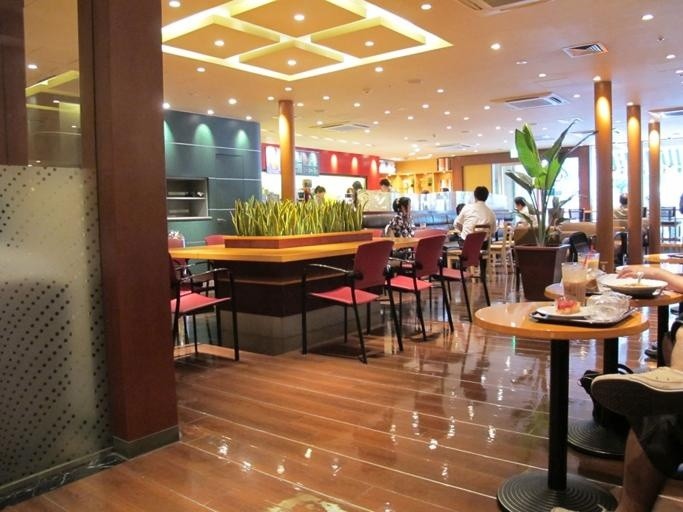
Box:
[562,262,588,305]
[578,253,599,278]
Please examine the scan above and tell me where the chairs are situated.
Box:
[167,238,209,298]
[168,252,240,361]
[366,234,454,341]
[613,232,628,266]
[570,232,591,262]
[490,226,514,274]
[301,240,404,365]
[416,232,490,322]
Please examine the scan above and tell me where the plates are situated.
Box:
[537,306,591,317]
[603,279,667,295]
[528,304,637,324]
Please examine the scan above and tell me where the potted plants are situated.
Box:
[506,118,598,300]
[223,194,373,248]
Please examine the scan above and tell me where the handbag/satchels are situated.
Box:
[576,363,634,432]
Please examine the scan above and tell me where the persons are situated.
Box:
[379,178,391,192]
[551,317,683,512]
[453,204,465,231]
[454,186,496,283]
[385,197,415,258]
[506,197,530,241]
[303,180,312,203]
[616,193,683,365]
[613,194,629,219]
[313,185,325,202]
[353,182,364,210]
